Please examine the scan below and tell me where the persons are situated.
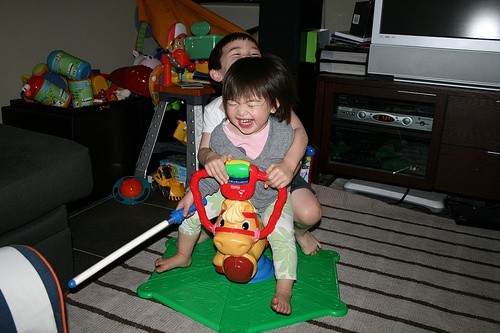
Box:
[153,57,298,315]
[176,33,322,256]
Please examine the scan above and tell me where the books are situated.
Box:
[318,31,371,76]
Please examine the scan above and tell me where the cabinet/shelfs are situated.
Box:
[313,73,500,208]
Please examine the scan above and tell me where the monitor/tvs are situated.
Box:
[368,0,500,92]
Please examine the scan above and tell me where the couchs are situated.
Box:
[0,124,93,293]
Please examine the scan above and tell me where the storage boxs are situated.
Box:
[2,99,188,214]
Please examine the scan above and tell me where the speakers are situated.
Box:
[350,2,374,38]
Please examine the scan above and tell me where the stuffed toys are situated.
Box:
[114,52,161,100]
